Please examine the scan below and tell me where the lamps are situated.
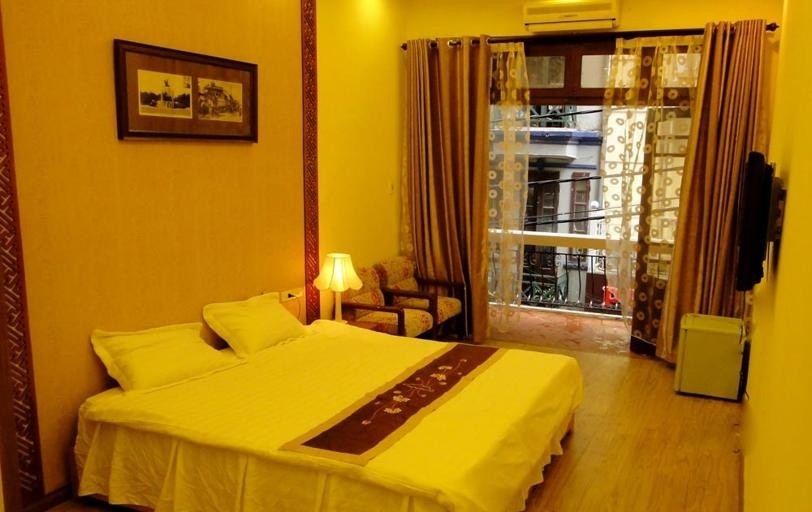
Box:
[316,253,362,326]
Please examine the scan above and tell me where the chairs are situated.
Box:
[332,256,469,339]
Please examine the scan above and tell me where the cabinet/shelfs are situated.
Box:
[674,314,744,402]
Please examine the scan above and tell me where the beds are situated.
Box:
[62,320,581,511]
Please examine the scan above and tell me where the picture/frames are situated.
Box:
[113,38,258,142]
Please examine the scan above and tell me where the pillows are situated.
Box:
[88,293,308,402]
